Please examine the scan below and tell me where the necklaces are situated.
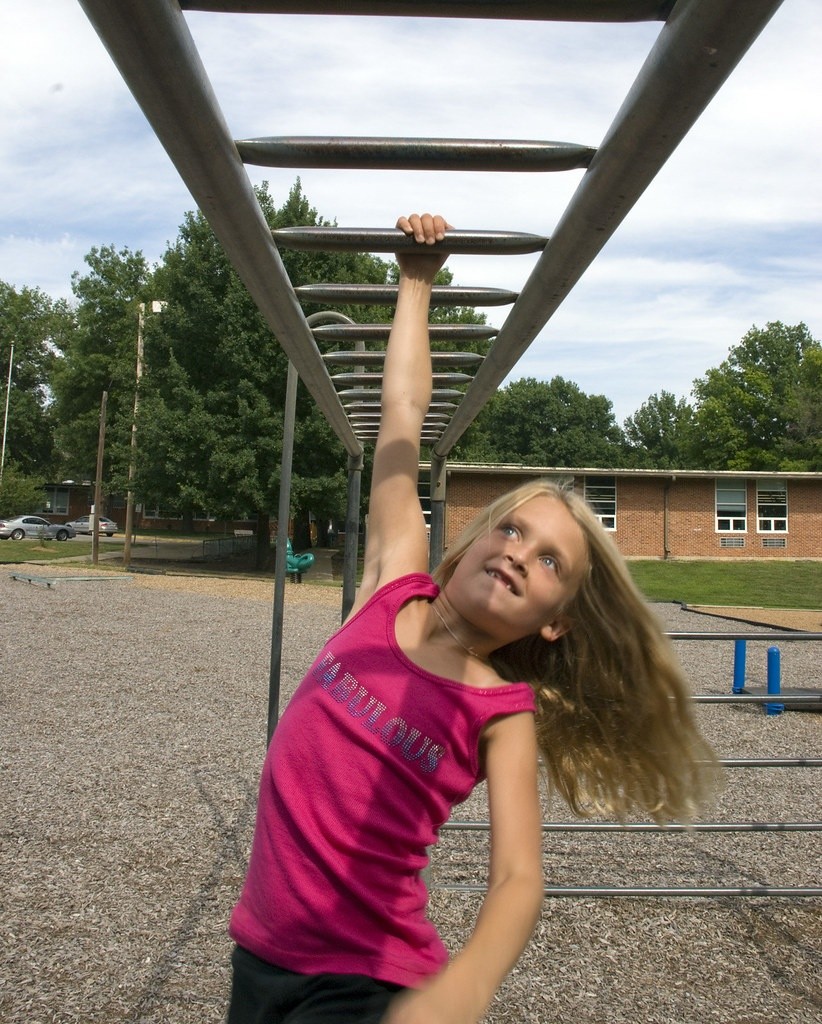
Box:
[432,604,490,662]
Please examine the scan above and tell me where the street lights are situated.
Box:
[122,301,170,564]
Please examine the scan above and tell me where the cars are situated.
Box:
[65,514,118,536]
[0,515,76,541]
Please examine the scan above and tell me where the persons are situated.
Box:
[225,214,726,1024]
